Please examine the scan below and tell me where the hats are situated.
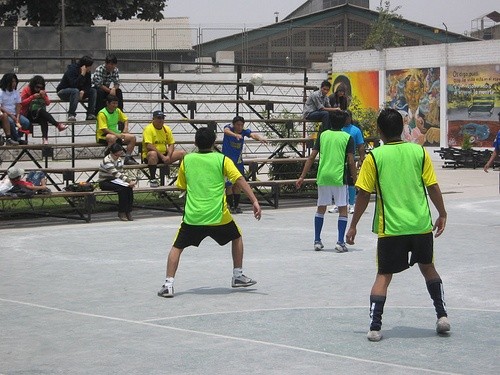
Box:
[336,85,345,92]
[153,110,165,117]
[8,166,24,179]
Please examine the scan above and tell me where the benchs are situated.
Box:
[0,80,377,222]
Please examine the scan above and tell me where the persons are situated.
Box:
[8,168,46,190]
[483,131,500,173]
[158,127,261,296]
[91,53,123,116]
[303,80,341,128]
[0,73,30,145]
[141,110,186,187]
[20,75,68,144]
[0,110,19,145]
[96,94,139,164]
[222,116,267,213]
[328,110,365,214]
[346,108,450,342]
[56,56,97,121]
[98,143,136,221]
[294,110,358,252]
[328,84,352,124]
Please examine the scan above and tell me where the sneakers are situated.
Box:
[335,242,346,250]
[124,156,139,165]
[232,275,257,287]
[314,240,324,251]
[157,284,174,297]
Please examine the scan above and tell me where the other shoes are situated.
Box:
[58,123,68,132]
[118,212,133,221]
[368,330,383,341]
[86,115,97,120]
[436,317,450,333]
[6,138,19,146]
[68,116,76,121]
[43,138,48,144]
[230,207,242,214]
[150,179,157,183]
